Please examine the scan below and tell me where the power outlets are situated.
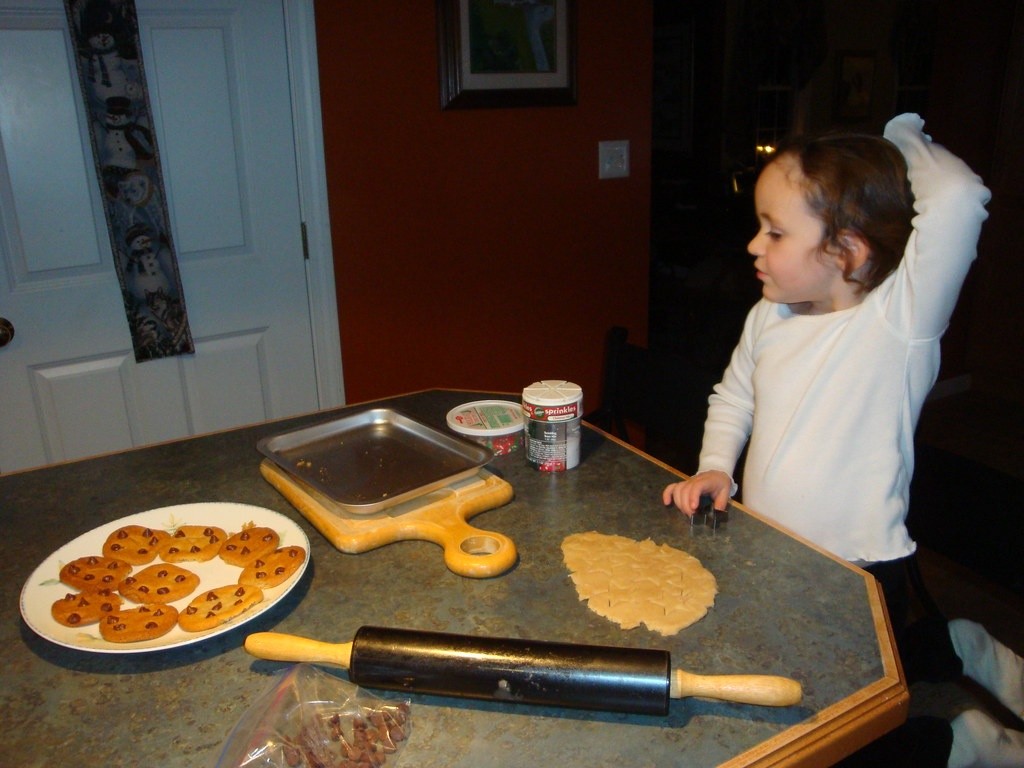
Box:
[597,140,631,180]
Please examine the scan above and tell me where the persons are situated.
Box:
[662,111,1024,768]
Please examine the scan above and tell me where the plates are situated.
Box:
[18,503,311,654]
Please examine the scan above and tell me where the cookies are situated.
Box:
[51,524,306,642]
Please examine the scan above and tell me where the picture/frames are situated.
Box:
[435,0,578,110]
[832,47,876,124]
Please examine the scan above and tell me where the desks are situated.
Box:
[0,389,909,768]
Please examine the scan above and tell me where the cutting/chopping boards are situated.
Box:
[261,448,518,577]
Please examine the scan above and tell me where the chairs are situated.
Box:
[582,326,725,475]
